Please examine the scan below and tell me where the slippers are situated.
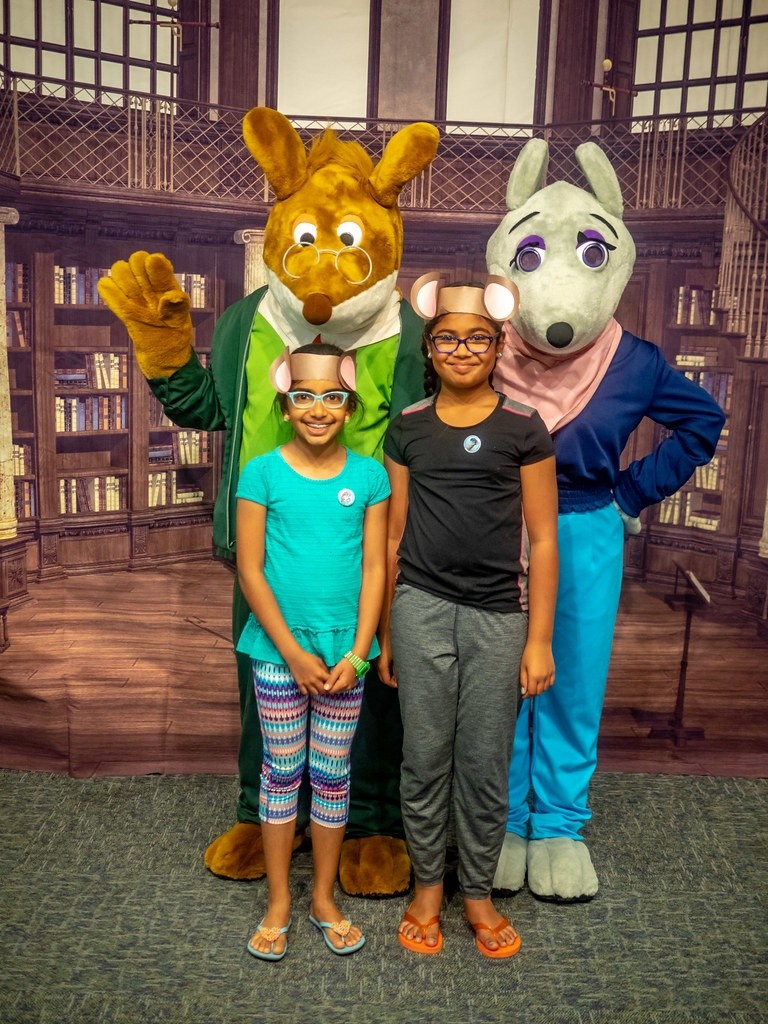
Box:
[472,915,522,959]
[398,899,443,955]
[308,914,365,954]
[247,917,292,960]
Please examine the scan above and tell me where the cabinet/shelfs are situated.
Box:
[5,230,221,585]
[640,256,756,602]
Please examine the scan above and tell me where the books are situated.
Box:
[3,262,734,531]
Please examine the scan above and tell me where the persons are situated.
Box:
[384,271,557,960]
[236,344,391,961]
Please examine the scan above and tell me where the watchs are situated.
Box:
[344,651,370,679]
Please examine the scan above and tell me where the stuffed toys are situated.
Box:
[485,137,725,903]
[95,105,439,896]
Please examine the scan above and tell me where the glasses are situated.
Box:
[428,332,500,354]
[285,391,350,410]
